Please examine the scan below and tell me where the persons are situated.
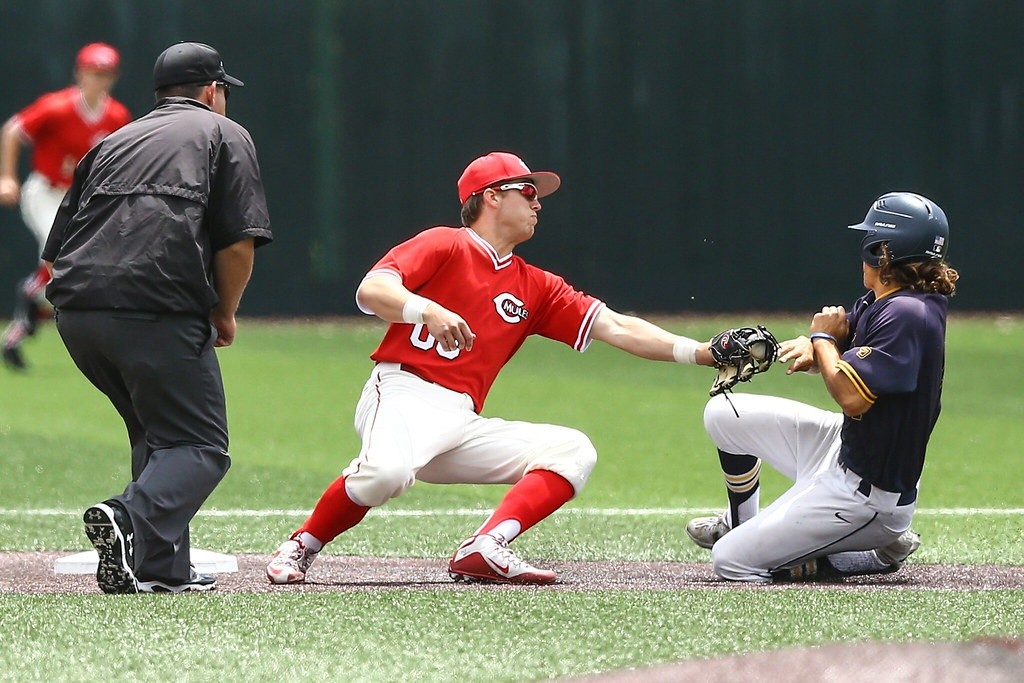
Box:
[0,42,134,373]
[686,193,958,584]
[40,40,272,596]
[263,152,783,586]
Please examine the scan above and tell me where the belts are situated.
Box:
[838,456,917,506]
[375,362,434,384]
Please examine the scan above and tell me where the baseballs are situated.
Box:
[751,339,767,359]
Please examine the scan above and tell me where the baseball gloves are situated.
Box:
[708,327,775,397]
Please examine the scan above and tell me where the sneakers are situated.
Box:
[83,501,138,594]
[133,562,217,593]
[447,532,557,584]
[265,533,319,583]
[685,510,731,550]
[868,529,922,569]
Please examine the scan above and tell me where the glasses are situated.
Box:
[471,183,538,203]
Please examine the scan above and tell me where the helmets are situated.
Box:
[847,191,949,269]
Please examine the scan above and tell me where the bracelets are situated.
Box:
[402,295,434,324]
[810,333,837,343]
[673,337,699,366]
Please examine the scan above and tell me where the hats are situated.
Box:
[152,40,245,91]
[457,152,560,206]
[78,42,120,74]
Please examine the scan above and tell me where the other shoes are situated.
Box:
[3,348,23,367]
[14,278,35,335]
